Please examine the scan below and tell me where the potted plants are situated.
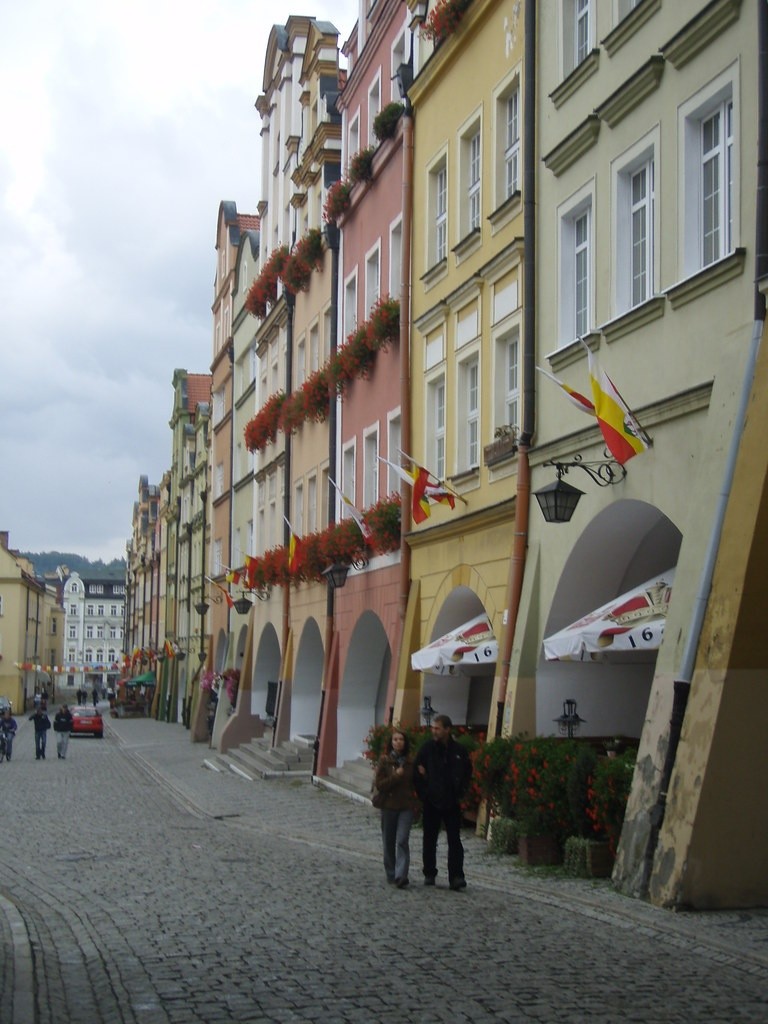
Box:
[604,740,617,758]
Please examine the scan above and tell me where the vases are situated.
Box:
[516,833,555,868]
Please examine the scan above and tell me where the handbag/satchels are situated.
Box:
[370,782,384,808]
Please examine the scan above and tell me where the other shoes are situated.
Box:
[58,752,61,758]
[396,876,409,888]
[450,874,466,890]
[35,756,40,760]
[387,874,395,884]
[40,752,45,759]
[424,874,436,885]
[61,756,65,759]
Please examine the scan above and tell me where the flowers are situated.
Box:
[371,102,404,142]
[362,716,638,876]
[243,491,403,593]
[417,0,470,45]
[244,224,327,321]
[243,294,400,453]
[200,671,216,689]
[343,143,376,184]
[222,668,240,683]
[322,179,352,225]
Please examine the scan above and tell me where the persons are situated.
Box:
[34,687,48,710]
[76,688,82,705]
[29,708,51,759]
[55,704,72,759]
[92,688,98,706]
[0,710,17,759]
[375,731,424,888]
[413,715,473,889]
[101,685,116,700]
[82,689,87,705]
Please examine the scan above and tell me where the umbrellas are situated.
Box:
[411,614,500,678]
[542,563,677,663]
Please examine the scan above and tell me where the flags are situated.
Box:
[119,640,180,673]
[339,491,382,549]
[587,349,648,465]
[550,376,596,416]
[213,532,328,609]
[386,459,456,524]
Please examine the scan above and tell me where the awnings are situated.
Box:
[125,671,156,687]
[118,676,132,688]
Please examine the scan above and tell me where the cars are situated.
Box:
[70,707,103,739]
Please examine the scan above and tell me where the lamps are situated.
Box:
[157,651,166,662]
[176,647,195,661]
[141,656,148,665]
[417,696,438,727]
[322,548,370,588]
[232,588,270,614]
[198,647,208,662]
[553,699,586,740]
[531,445,627,523]
[194,594,223,613]
